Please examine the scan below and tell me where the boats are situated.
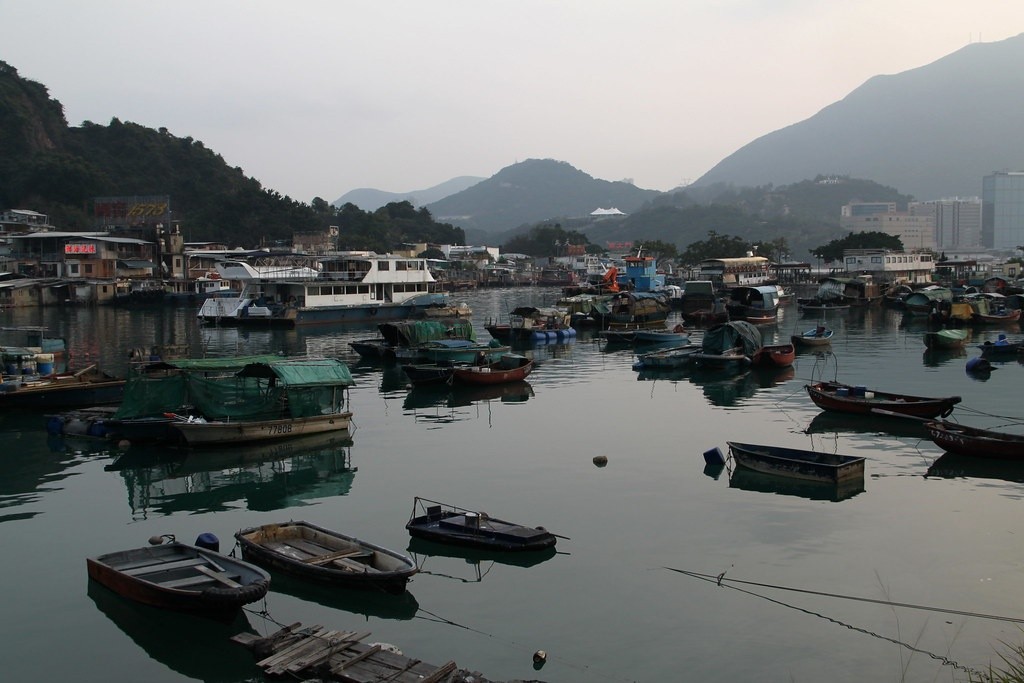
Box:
[103,353,286,443]
[446,352,535,385]
[790,325,834,346]
[632,344,704,372]
[725,286,781,323]
[923,328,973,349]
[924,419,1024,461]
[85,533,272,618]
[688,320,795,378]
[632,329,691,340]
[483,306,576,343]
[167,359,357,446]
[806,380,962,419]
[347,318,512,388]
[727,441,866,482]
[234,520,418,594]
[406,497,571,552]
[555,280,727,341]
[197,253,449,327]
[796,275,1024,325]
[0,365,163,409]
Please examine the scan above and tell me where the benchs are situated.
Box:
[158,570,241,588]
[119,556,214,575]
[303,548,360,563]
